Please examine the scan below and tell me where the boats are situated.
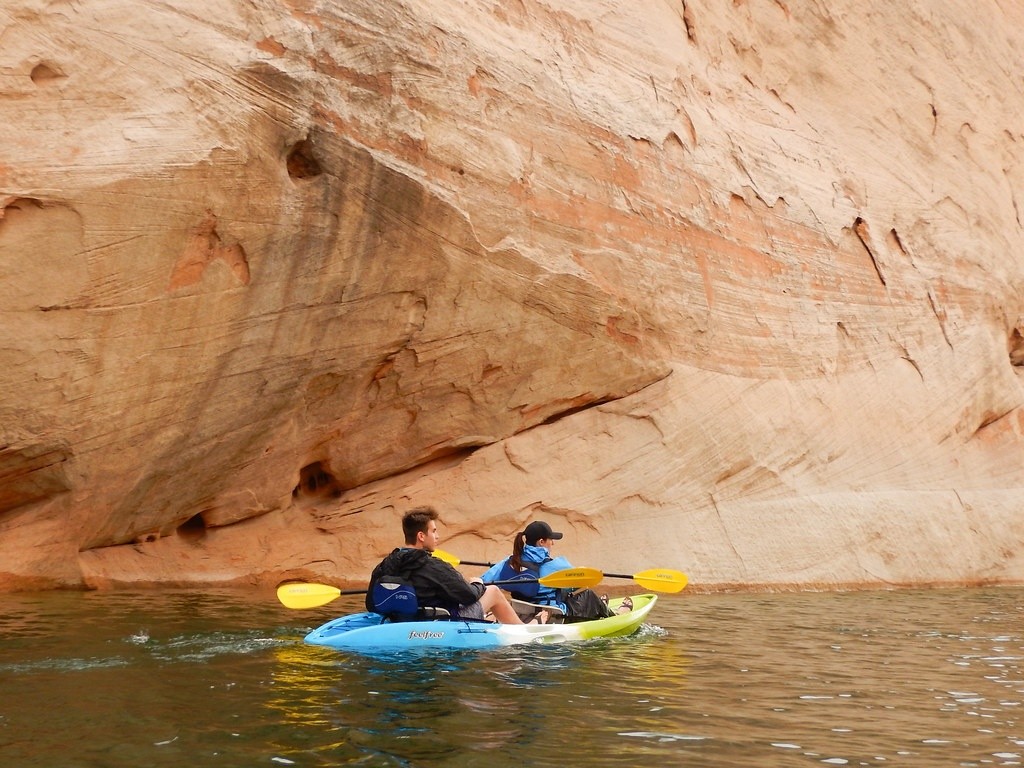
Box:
[301,593,660,655]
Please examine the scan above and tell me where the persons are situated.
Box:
[480,521,633,625]
[366,505,552,624]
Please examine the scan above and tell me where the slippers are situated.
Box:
[533,609,553,624]
[599,593,609,606]
[618,597,633,612]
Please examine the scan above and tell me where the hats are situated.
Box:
[525,521,563,540]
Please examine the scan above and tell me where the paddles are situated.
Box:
[276,567,604,610]
[431,549,689,594]
